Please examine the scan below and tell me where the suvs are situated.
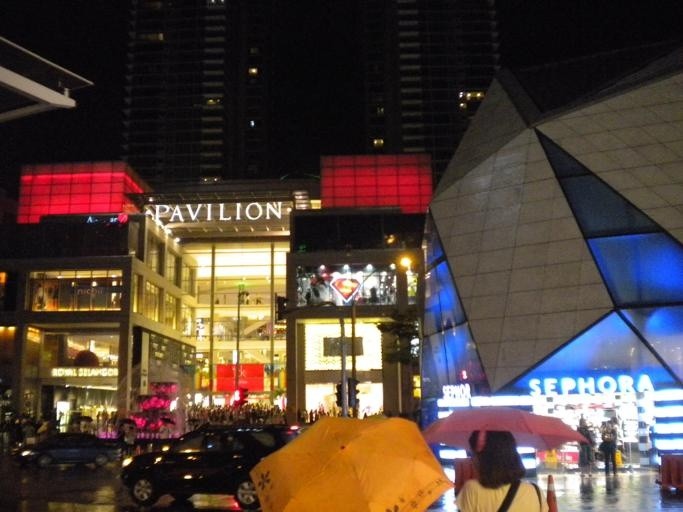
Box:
[120,424,312,510]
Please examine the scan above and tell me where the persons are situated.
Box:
[578,414,621,475]
[34,284,48,310]
[456,433,549,512]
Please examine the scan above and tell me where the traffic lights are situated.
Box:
[239,387,248,405]
[348,379,360,407]
[334,383,343,407]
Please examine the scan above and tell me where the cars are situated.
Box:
[11,432,118,470]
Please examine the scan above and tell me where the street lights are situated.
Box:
[351,257,411,419]
[235,291,249,422]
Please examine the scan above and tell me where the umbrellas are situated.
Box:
[419,403,596,461]
[250,414,454,512]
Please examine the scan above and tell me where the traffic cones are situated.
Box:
[546,474,558,512]
